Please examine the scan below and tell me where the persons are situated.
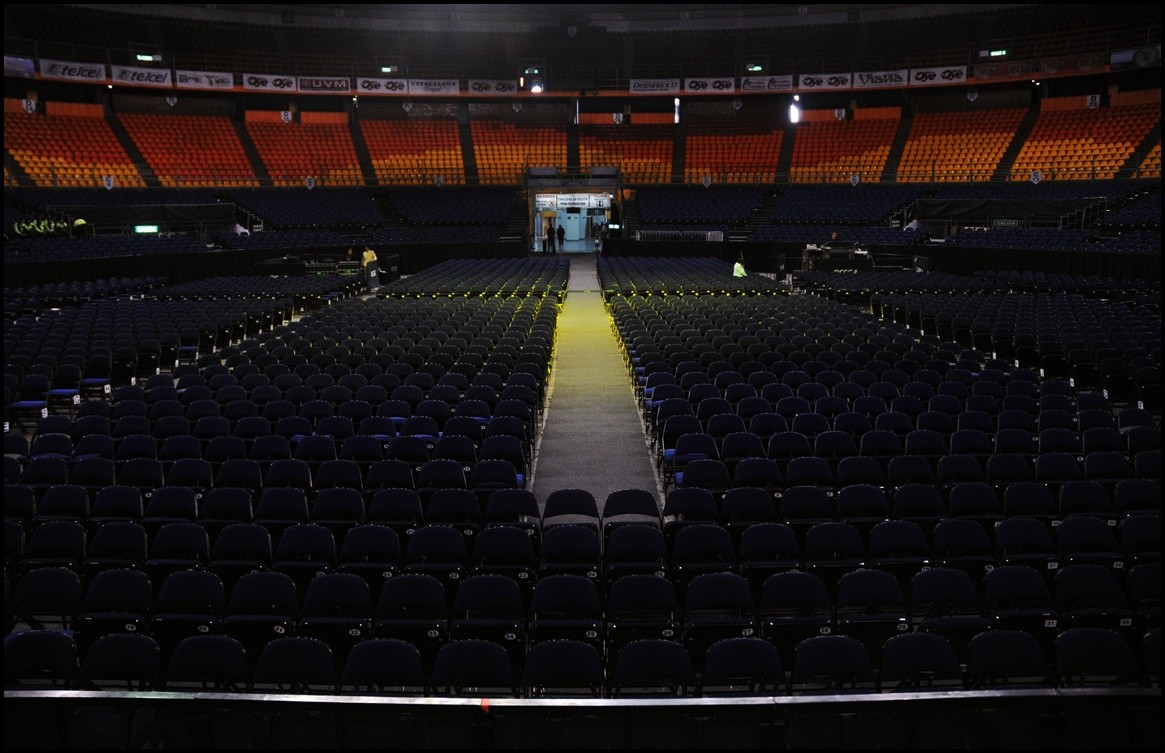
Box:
[829,232,839,241]
[12,214,87,239]
[594,222,604,232]
[361,246,377,280]
[547,224,565,246]
[733,259,747,277]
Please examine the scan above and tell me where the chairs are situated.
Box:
[0,101,1165,697]
